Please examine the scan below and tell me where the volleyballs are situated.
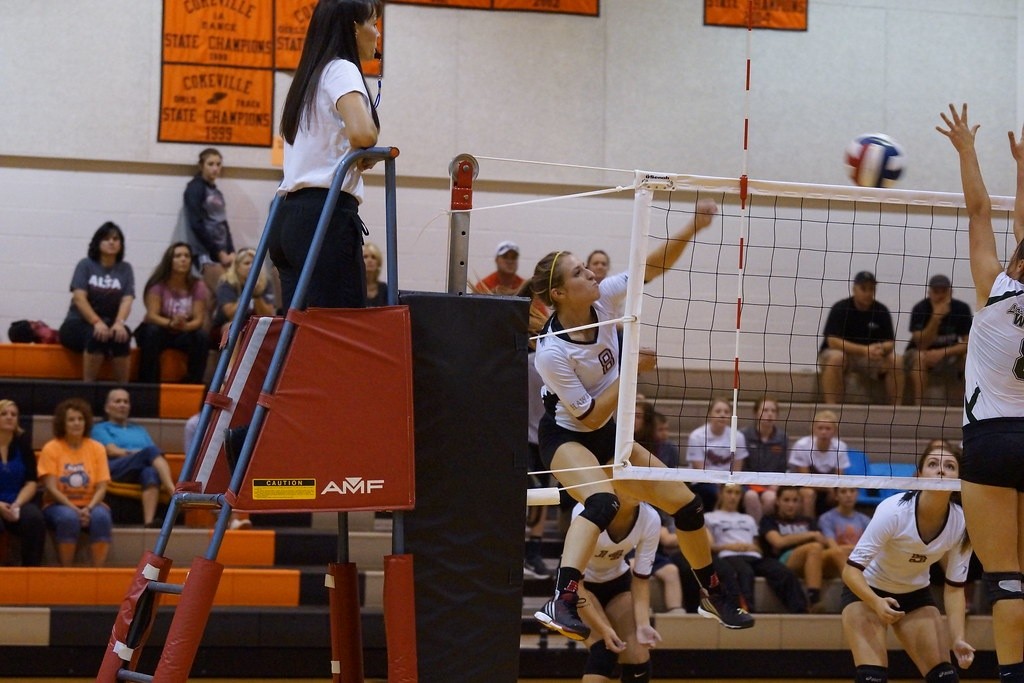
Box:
[843,133,905,188]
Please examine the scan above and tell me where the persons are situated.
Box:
[903,275,973,406]
[36,398,112,567]
[58,221,137,384]
[269,0,380,309]
[359,243,389,308]
[571,490,664,683]
[0,399,45,567]
[838,445,975,683]
[520,353,556,580]
[585,250,624,376]
[936,102,1024,683]
[631,391,870,617]
[89,388,176,529]
[185,377,226,458]
[210,247,285,352]
[518,199,758,641]
[183,149,236,297]
[470,241,552,353]
[818,271,905,407]
[133,242,211,384]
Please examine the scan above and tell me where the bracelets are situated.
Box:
[85,506,92,514]
[168,319,173,328]
[91,317,102,326]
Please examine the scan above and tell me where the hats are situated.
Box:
[496,241,520,256]
[854,271,877,285]
[929,275,951,289]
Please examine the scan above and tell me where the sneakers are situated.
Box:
[534,593,591,640]
[698,595,754,629]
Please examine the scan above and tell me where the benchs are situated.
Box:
[0,320,996,653]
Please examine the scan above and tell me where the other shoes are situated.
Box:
[523,557,553,579]
